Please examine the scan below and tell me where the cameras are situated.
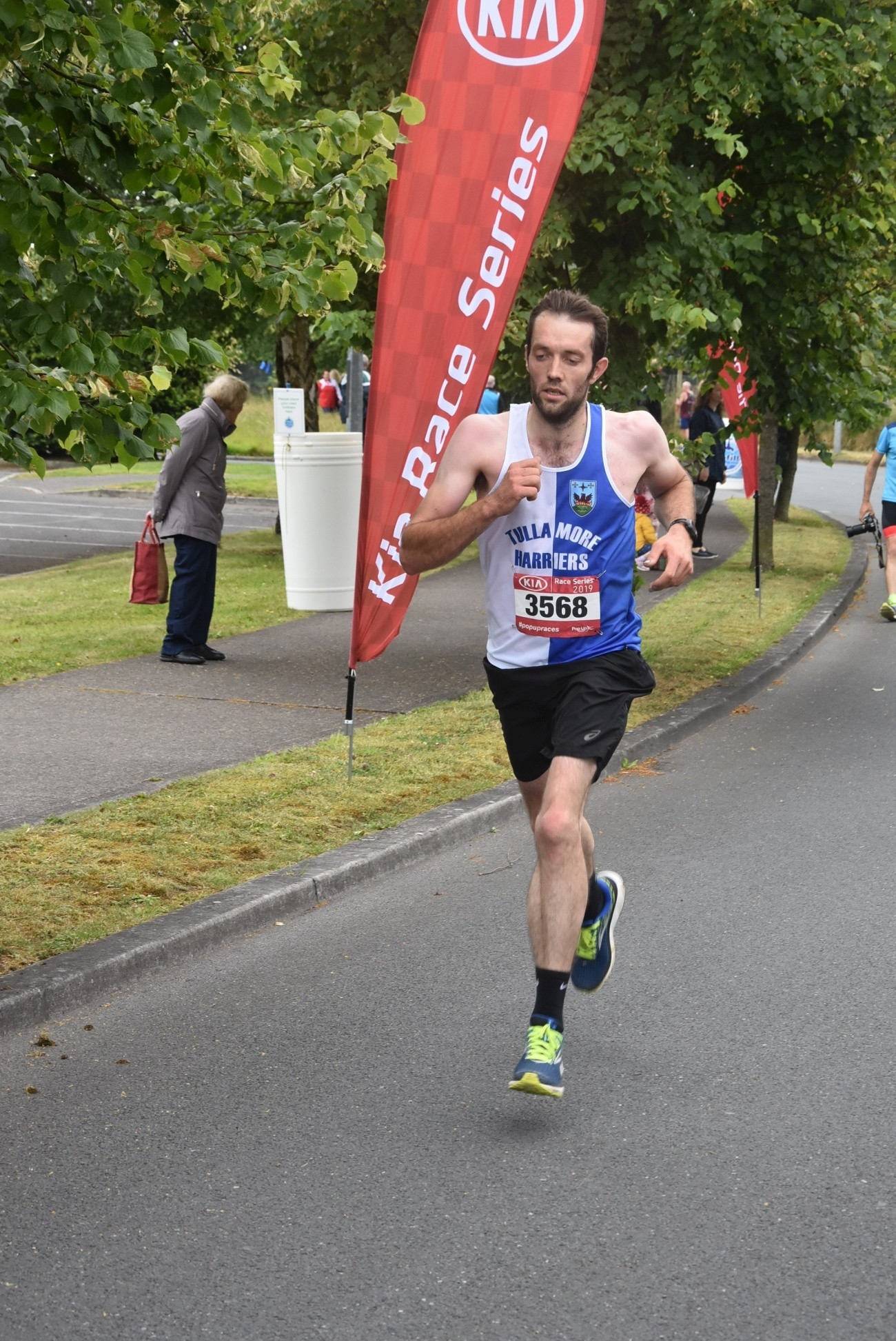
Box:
[846,515,876,538]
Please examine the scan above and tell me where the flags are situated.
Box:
[707,337,758,499]
[350,0,604,672]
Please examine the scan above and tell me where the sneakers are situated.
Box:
[880,603,896,623]
[571,871,625,992]
[507,1024,566,1096]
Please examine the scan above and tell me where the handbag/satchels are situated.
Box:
[129,515,169,604]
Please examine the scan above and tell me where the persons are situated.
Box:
[316,354,505,424]
[143,375,249,666]
[634,381,738,572]
[399,291,694,1105]
[858,421,896,622]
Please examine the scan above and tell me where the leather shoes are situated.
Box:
[159,649,206,664]
[191,643,226,661]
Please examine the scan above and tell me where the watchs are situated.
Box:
[669,518,698,542]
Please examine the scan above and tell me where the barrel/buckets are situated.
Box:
[275,431,365,611]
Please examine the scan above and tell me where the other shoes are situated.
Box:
[692,549,718,559]
